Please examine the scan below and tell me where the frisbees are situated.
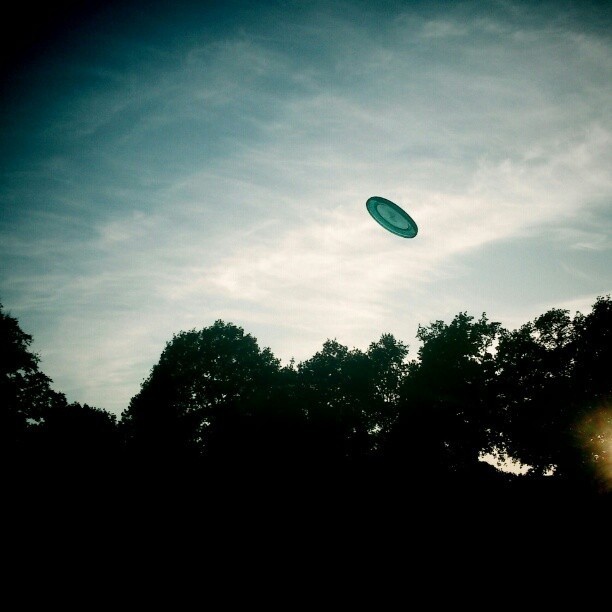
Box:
[366,196,417,238]
